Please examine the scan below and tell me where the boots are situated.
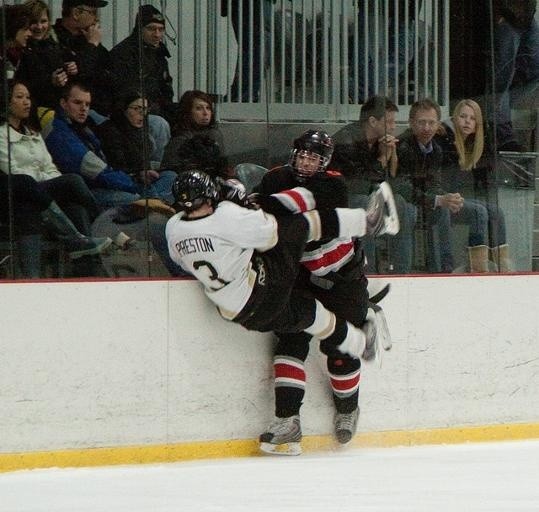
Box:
[39,200,112,259]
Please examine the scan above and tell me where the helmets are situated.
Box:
[288,129,335,182]
[172,170,219,206]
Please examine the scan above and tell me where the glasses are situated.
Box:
[141,25,165,32]
[76,8,97,16]
[128,105,151,112]
[409,118,442,127]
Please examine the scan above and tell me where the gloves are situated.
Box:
[223,177,246,206]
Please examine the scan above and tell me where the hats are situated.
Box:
[135,4,165,31]
[62,0,108,9]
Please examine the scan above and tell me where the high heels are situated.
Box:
[112,233,136,251]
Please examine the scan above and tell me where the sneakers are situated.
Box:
[362,309,376,361]
[335,407,359,443]
[366,188,386,240]
[259,415,301,445]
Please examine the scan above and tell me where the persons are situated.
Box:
[0,0,539,279]
[164,167,392,366]
[246,128,363,447]
